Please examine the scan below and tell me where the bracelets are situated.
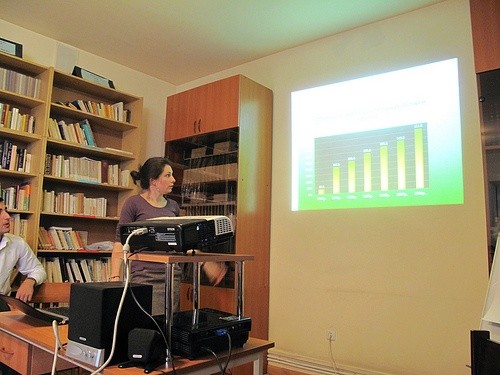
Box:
[200,262,206,268]
[110,276,119,279]
[26,277,37,286]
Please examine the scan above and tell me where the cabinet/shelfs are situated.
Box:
[0,49,144,309]
[164,74,274,375]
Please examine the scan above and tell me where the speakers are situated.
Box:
[118,327,166,374]
[65,281,153,368]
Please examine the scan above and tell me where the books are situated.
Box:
[0,182,32,211]
[37,226,88,250]
[43,189,107,217]
[191,141,236,158]
[8,214,28,242]
[37,257,112,283]
[180,155,238,185]
[184,205,234,215]
[0,139,31,172]
[44,153,131,188]
[0,102,35,135]
[10,267,26,287]
[48,119,134,156]
[182,184,232,203]
[27,302,72,309]
[0,66,41,100]
[53,98,131,124]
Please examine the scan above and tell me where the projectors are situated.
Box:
[120,215,235,254]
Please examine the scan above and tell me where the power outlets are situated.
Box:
[326,330,336,341]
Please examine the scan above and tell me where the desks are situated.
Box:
[0,310,275,375]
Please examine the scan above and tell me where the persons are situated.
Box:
[0,197,46,312]
[110,157,226,316]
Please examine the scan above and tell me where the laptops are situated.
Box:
[0,294,70,324]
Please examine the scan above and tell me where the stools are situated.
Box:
[121,251,255,369]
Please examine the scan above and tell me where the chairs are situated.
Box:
[20,280,80,309]
